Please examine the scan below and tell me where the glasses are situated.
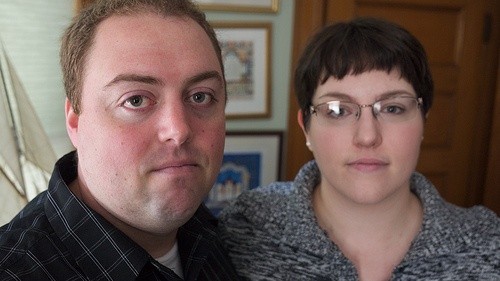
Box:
[309,96,422,126]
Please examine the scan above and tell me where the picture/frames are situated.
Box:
[204,21,273,119]
[204,129,285,216]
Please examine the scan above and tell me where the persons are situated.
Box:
[210,16,500,281]
[0,0,241,281]
